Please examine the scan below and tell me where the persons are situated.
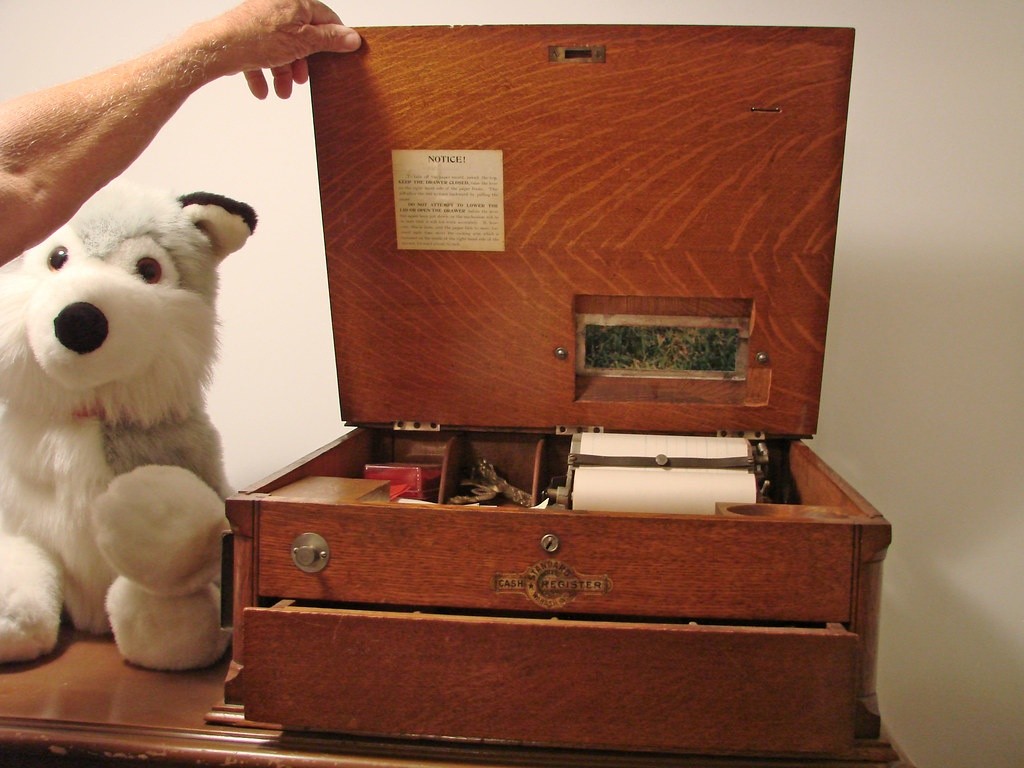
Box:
[0,0,362,268]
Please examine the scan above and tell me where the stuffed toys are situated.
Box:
[0,190,258,671]
